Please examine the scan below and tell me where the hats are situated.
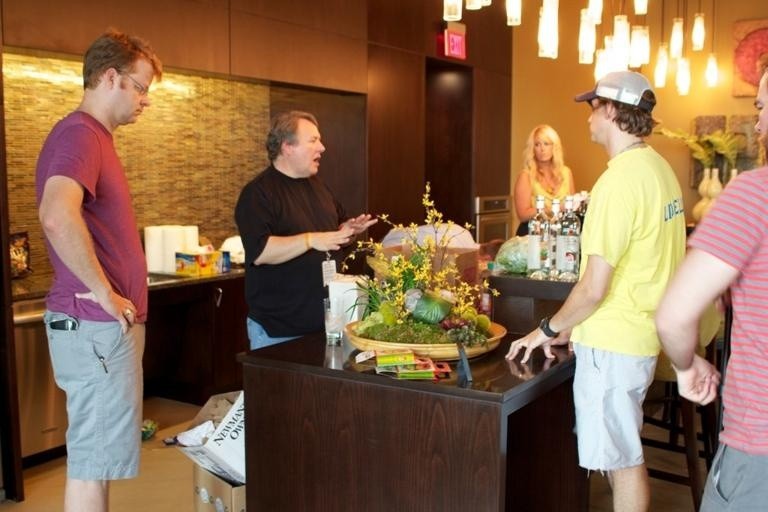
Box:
[575,71,656,111]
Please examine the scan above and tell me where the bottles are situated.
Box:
[529,195,552,273]
[557,196,580,274]
[549,199,562,268]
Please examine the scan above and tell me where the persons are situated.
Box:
[34,30,163,512]
[657,55,763,511]
[514,123,576,241]
[234,111,377,348]
[505,72,686,511]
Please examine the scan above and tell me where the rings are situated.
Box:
[123,309,132,317]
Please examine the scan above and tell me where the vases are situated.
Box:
[702,168,723,225]
[343,319,507,361]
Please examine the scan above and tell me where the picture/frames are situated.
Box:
[729,18,768,98]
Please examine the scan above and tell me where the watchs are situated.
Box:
[538,312,560,339]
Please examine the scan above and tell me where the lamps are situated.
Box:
[654,45,671,89]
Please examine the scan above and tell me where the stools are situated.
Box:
[639,332,723,511]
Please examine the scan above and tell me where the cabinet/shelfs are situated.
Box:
[10,299,68,467]
[140,277,254,405]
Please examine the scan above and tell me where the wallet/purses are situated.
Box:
[51,319,76,332]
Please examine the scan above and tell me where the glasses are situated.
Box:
[117,70,149,95]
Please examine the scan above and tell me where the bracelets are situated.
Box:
[305,231,311,253]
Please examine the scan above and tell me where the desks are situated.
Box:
[480,270,580,344]
[240,328,594,511]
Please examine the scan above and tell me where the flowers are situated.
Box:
[323,178,507,349]
